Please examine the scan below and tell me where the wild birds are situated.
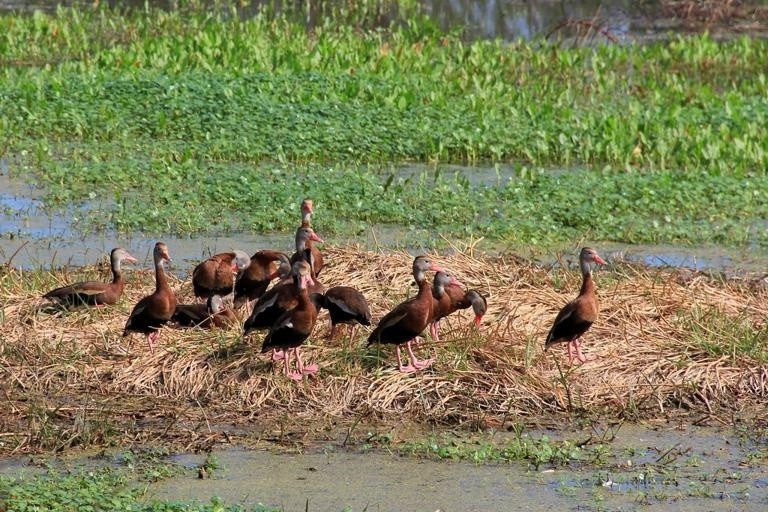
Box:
[365,255,487,374]
[544,246,609,367]
[120,242,177,358]
[308,286,372,341]
[171,199,325,383]
[41,246,137,312]
